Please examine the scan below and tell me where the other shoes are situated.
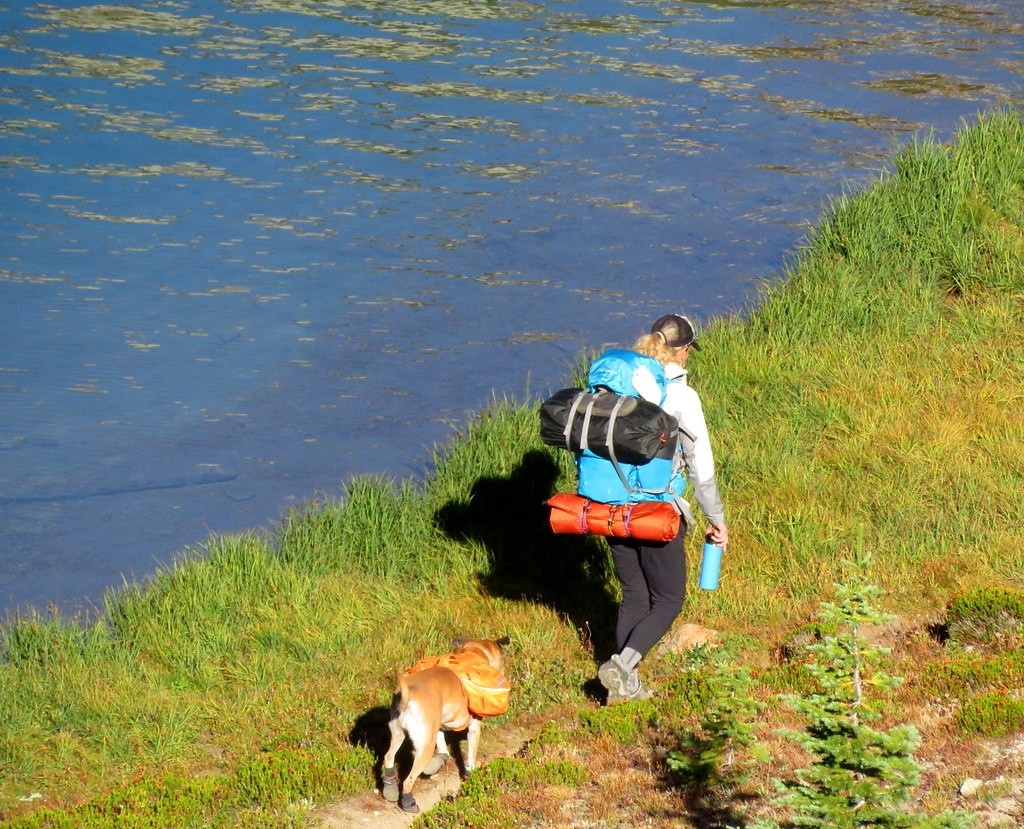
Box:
[598,654,652,707]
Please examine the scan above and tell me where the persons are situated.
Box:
[573,315,730,705]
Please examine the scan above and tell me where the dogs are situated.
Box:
[380,634,511,815]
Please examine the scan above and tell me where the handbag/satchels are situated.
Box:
[397,652,512,717]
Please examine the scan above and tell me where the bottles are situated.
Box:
[699,534,722,590]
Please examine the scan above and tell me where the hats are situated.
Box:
[652,313,702,352]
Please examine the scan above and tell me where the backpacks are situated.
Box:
[576,346,687,504]
